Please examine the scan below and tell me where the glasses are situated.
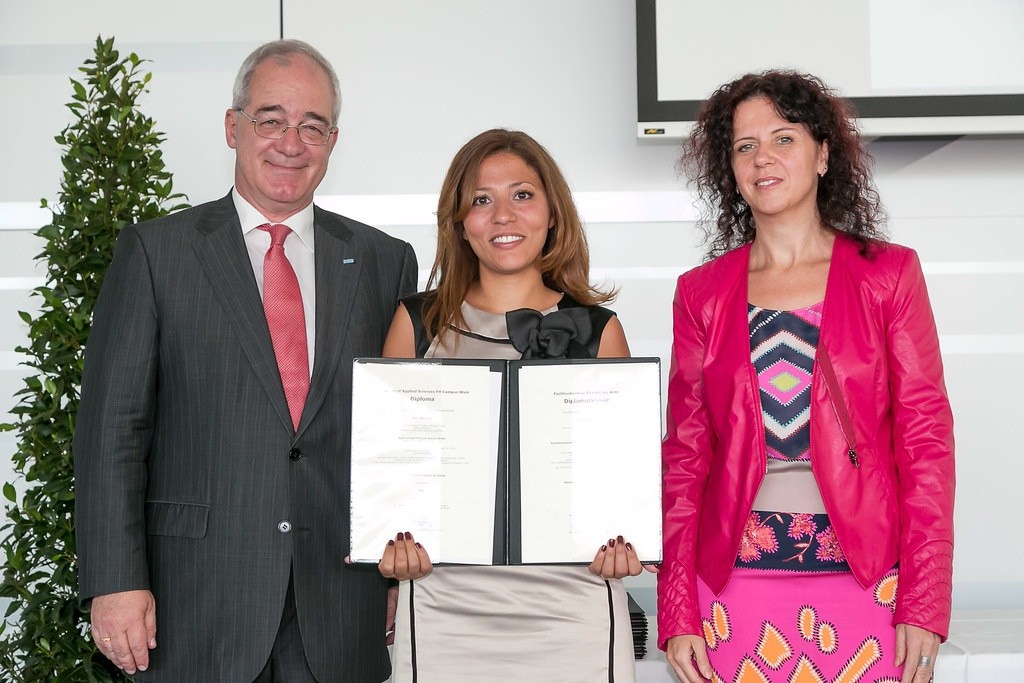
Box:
[236,107,336,146]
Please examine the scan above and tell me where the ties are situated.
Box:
[255,223,311,433]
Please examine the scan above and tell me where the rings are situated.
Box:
[601,575,610,580]
[919,656,930,666]
[101,638,110,641]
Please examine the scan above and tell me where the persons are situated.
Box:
[76,39,419,683]
[656,73,956,683]
[377,128,660,683]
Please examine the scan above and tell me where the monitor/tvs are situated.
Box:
[636,1,1024,139]
